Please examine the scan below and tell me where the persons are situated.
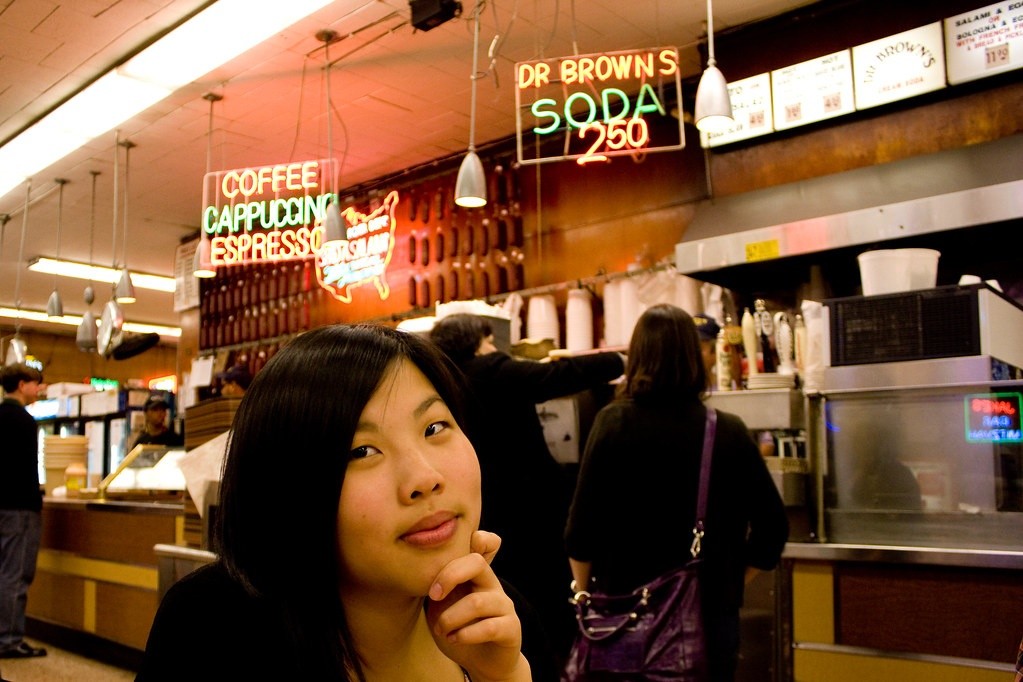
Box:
[133,322,560,682]
[130,393,185,462]
[218,363,253,400]
[563,303,790,682]
[425,313,628,682]
[0,362,48,659]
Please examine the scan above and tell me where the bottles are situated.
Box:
[715,329,733,390]
[726,313,742,388]
[742,300,808,373]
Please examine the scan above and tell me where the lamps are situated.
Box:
[190,91,224,280]
[314,29,350,255]
[693,0,735,132]
[0,129,182,364]
[453,0,488,208]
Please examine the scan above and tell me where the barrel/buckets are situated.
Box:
[44,437,88,496]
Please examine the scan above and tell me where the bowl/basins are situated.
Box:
[858,249,940,296]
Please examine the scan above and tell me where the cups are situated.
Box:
[524,267,702,351]
[64,464,85,491]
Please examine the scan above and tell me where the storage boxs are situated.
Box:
[700,389,803,510]
[25,381,154,422]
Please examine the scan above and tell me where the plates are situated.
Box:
[747,374,795,390]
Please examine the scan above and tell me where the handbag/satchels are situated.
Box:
[560,560,717,682]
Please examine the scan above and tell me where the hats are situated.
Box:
[692,314,721,339]
[142,395,171,412]
[217,367,254,387]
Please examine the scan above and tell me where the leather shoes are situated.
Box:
[0,643,46,658]
